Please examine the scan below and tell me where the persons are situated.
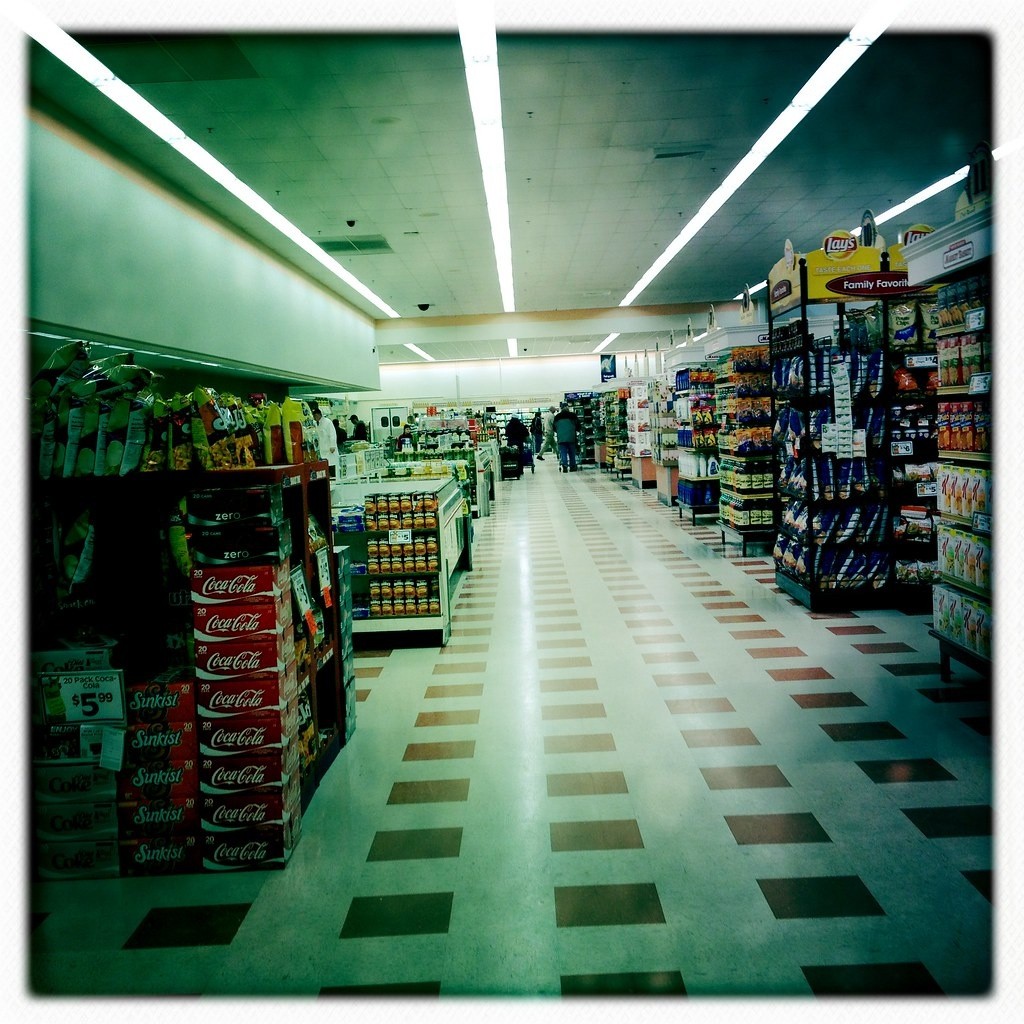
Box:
[552,403,582,472]
[505,413,529,475]
[534,411,545,454]
[537,406,559,461]
[398,424,413,451]
[347,415,368,442]
[333,419,347,451]
[312,409,340,477]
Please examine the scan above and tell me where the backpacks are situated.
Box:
[530,417,537,432]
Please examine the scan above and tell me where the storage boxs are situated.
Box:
[198,735,299,796]
[191,557,292,605]
[120,833,203,877]
[194,591,292,644]
[196,695,299,756]
[194,625,296,680]
[124,668,196,725]
[115,760,198,804]
[201,769,301,830]
[186,482,284,528]
[186,520,293,565]
[122,720,197,763]
[202,804,303,874]
[117,796,201,839]
[193,659,298,718]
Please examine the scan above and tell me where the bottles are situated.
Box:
[678,451,720,478]
[392,448,477,506]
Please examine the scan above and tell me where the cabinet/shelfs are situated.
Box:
[26,144,995,885]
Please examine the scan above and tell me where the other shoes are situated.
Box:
[563,467,567,472]
[537,455,545,460]
[570,467,577,472]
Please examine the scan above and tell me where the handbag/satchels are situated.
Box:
[521,449,532,463]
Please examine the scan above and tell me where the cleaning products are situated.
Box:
[677,479,716,506]
[677,450,722,478]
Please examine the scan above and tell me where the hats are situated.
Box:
[561,403,568,409]
[550,406,557,412]
[512,414,519,418]
